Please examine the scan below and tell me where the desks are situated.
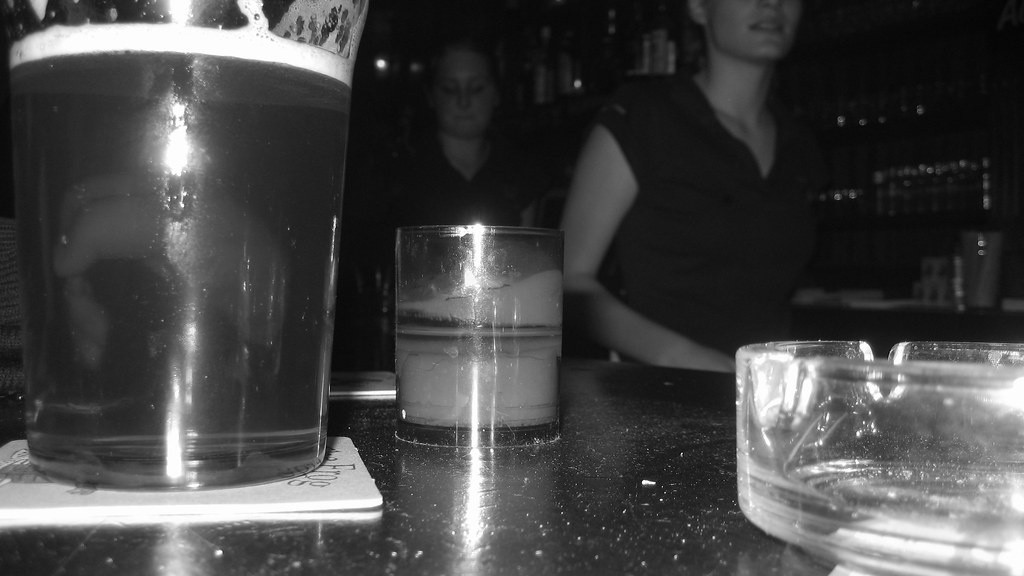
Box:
[0,359,1024,576]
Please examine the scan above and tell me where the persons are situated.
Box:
[557,1,883,373]
[342,39,538,372]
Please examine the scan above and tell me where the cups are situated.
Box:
[0,0,370,494]
[395,223,568,450]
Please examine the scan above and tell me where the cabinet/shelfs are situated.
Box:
[481,0,1024,340]
